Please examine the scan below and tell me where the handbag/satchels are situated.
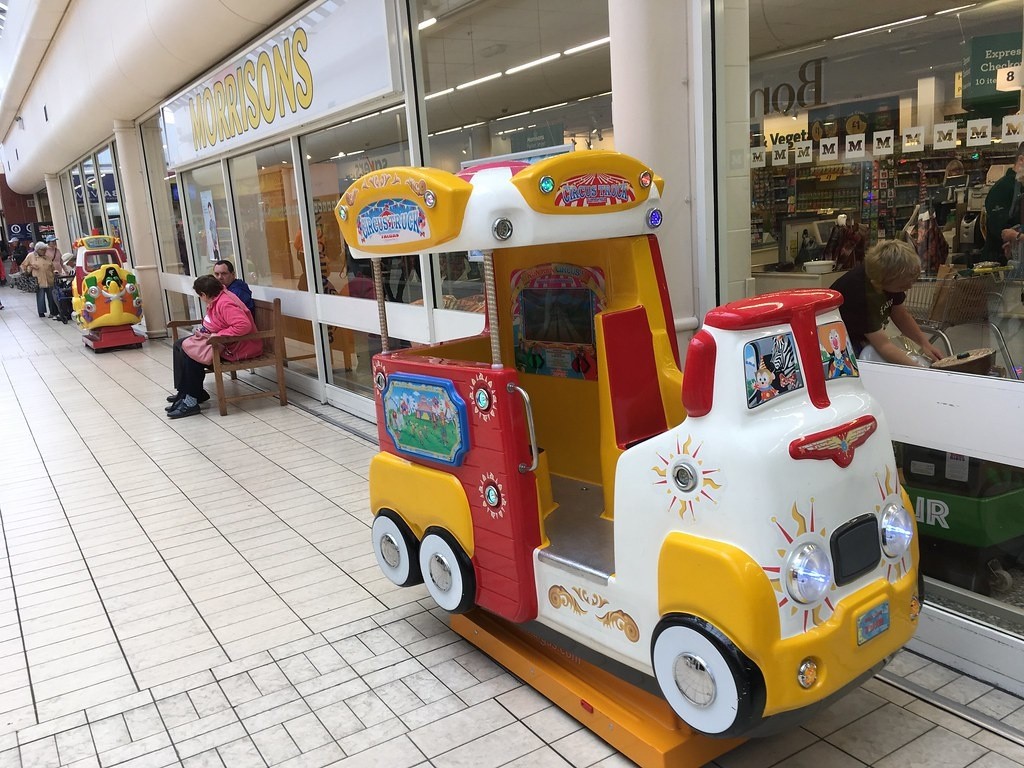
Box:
[181,328,227,366]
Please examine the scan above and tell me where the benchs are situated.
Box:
[165,293,287,417]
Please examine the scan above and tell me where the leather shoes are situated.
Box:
[165,399,201,418]
[166,389,210,404]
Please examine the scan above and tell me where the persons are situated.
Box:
[982,143,1024,267]
[205,202,219,261]
[298,253,339,297]
[339,263,412,376]
[44,235,69,317]
[53,253,76,321]
[166,260,255,402]
[293,226,306,278]
[19,241,55,318]
[831,240,945,369]
[7,237,27,272]
[8,242,18,275]
[165,274,264,420]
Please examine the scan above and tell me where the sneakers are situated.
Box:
[0,304,5,310]
[39,313,45,317]
[47,314,54,318]
[52,315,57,320]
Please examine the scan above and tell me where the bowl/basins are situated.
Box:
[804,261,835,274]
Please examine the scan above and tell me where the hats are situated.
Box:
[7,237,19,243]
[46,235,59,242]
[60,252,74,266]
